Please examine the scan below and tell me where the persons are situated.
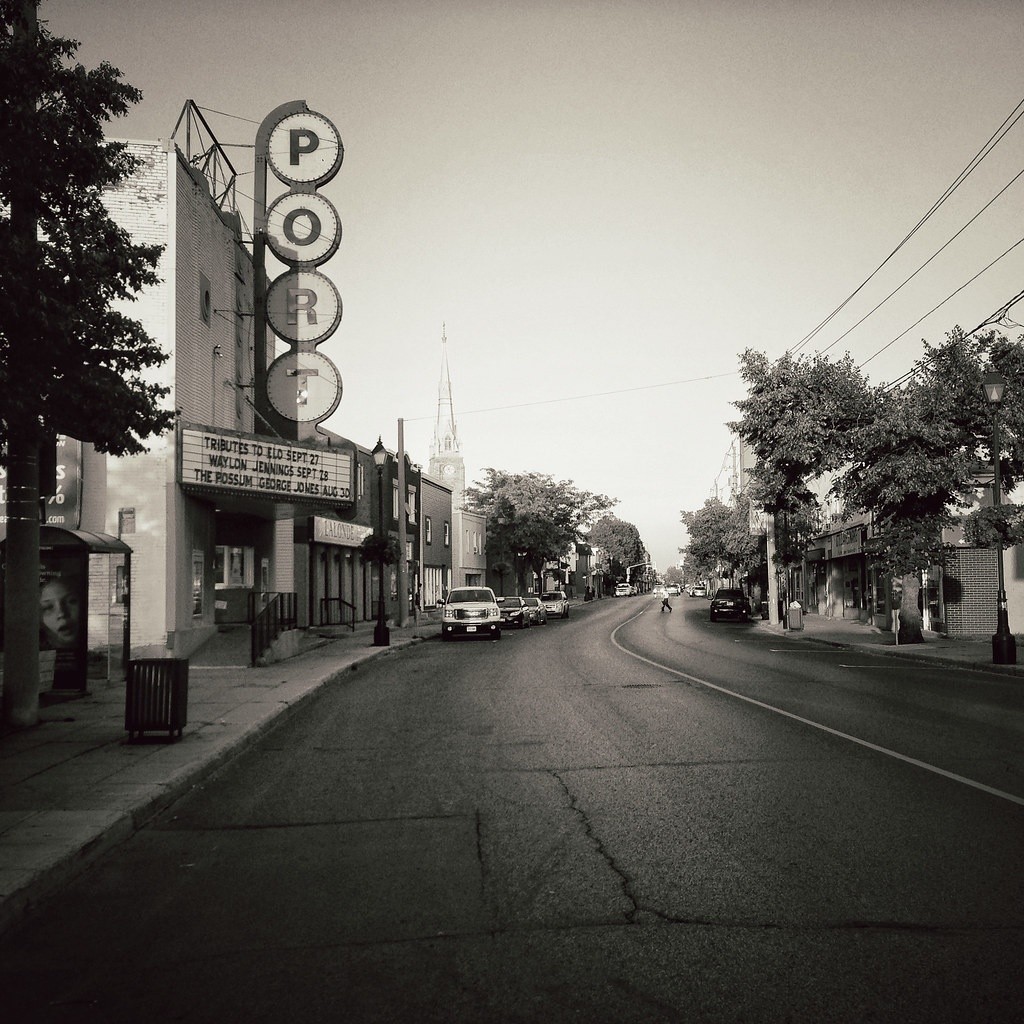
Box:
[661,589,672,612]
[39,577,79,645]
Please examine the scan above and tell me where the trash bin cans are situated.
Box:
[788,608,803,630]
[121,655,190,739]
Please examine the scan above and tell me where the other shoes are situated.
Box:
[661,610,664,612]
[669,609,672,612]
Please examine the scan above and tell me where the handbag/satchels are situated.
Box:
[659,601,664,608]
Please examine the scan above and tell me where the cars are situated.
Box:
[496,595,532,628]
[612,581,707,597]
[523,598,549,626]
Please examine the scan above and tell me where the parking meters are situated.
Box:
[891,591,901,646]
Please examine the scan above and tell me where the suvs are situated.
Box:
[708,587,750,622]
[538,590,571,620]
[435,584,504,640]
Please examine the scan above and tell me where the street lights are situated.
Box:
[495,512,510,596]
[981,370,1017,665]
[370,434,391,646]
